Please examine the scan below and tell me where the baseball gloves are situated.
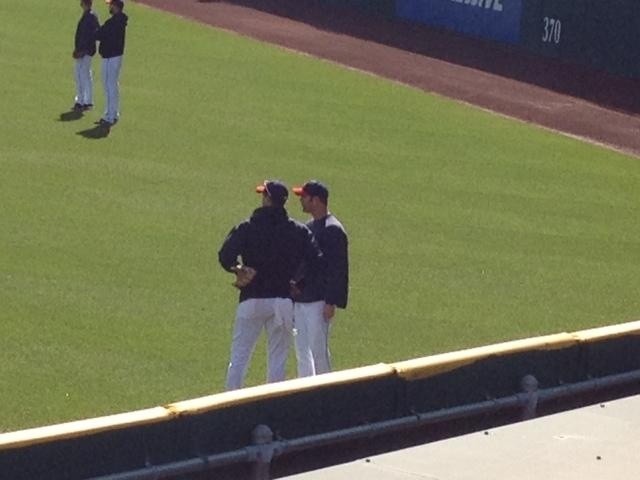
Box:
[233,265,257,290]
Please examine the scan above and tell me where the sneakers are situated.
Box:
[71,103,118,125]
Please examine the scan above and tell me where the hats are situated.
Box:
[292,180,328,203]
[254,180,289,205]
[106,0,124,8]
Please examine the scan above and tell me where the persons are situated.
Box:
[216,178,322,390]
[290,180,351,380]
[70,0,101,111]
[92,0,129,126]
